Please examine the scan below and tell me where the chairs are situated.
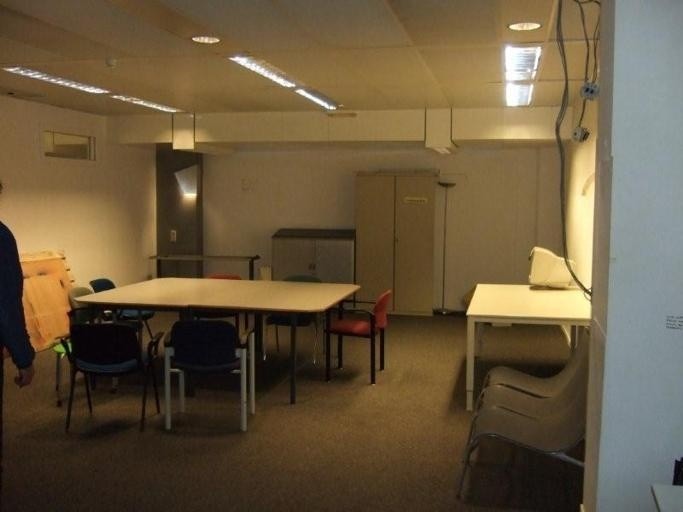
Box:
[323,285,397,389]
[53,278,159,437]
[456,324,591,499]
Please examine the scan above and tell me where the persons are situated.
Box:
[0,183,36,388]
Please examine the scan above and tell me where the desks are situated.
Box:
[464,282,593,414]
[72,277,362,432]
[148,251,261,280]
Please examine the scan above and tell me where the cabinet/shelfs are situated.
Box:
[271,228,356,312]
[354,170,437,318]
